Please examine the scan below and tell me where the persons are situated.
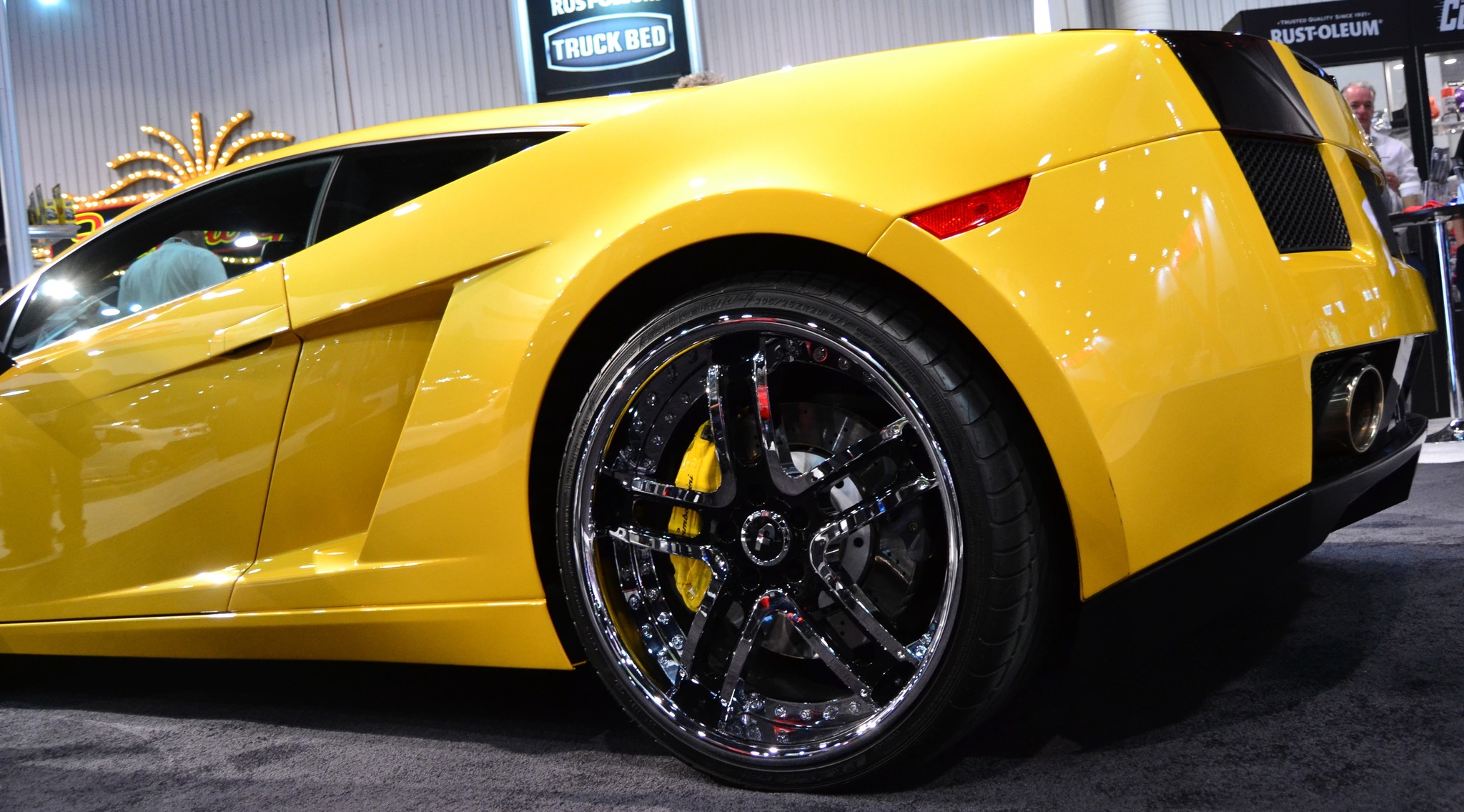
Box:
[1343,82,1419,210]
[33,228,226,348]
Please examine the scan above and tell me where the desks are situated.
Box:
[1387,204,1464,443]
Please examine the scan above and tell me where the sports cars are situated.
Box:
[3,26,1440,799]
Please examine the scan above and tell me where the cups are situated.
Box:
[1398,181,1420,208]
